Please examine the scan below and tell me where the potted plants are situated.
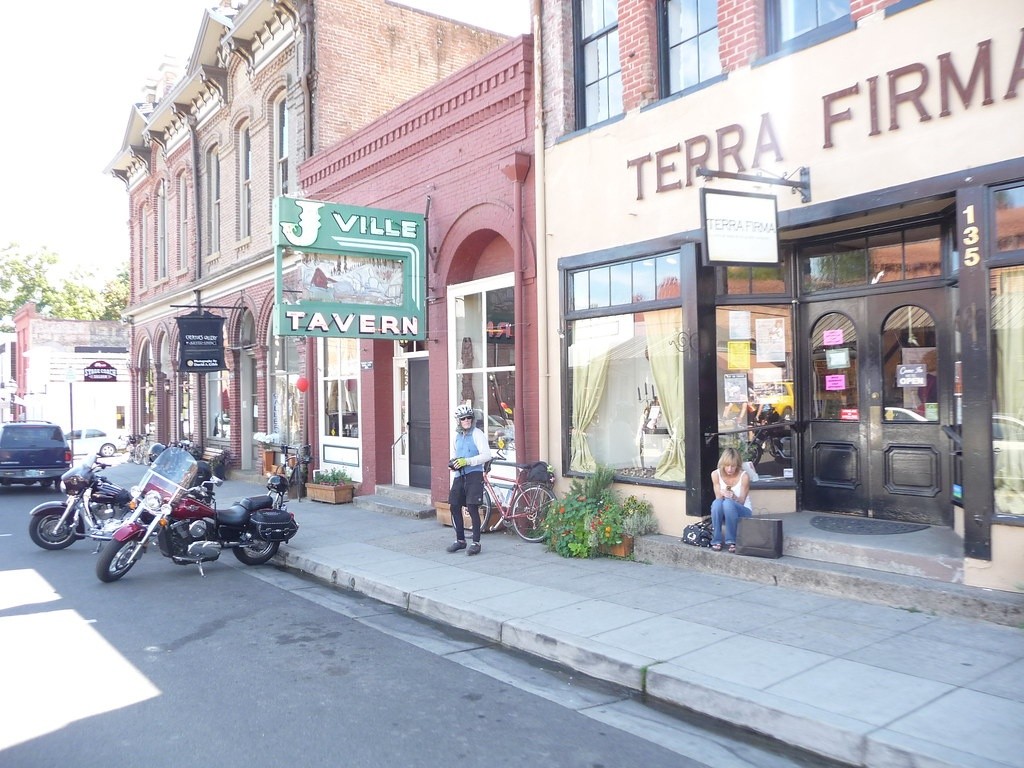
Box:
[559,467,655,558]
[305,469,354,505]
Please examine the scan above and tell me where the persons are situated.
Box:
[710,448,751,552]
[723,383,777,450]
[446,404,492,554]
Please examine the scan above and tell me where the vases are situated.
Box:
[262,442,298,454]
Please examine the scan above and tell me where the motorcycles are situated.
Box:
[28,444,213,551]
[737,402,793,464]
[96,447,300,583]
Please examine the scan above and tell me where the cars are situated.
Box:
[752,379,796,420]
[63,426,127,457]
[467,407,517,446]
[344,422,358,438]
[991,414,1024,491]
[884,405,937,423]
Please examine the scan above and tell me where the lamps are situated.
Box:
[399,339,408,348]
[149,383,157,396]
[163,379,173,395]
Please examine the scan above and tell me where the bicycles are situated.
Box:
[451,447,557,542]
[133,432,151,466]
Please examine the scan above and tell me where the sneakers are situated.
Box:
[446,539,467,552]
[465,543,481,556]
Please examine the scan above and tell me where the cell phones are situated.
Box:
[726,485,731,492]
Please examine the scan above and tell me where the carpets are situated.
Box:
[810,514,929,535]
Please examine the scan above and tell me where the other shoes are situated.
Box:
[728,544,736,552]
[712,544,722,551]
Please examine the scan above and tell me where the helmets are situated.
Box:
[455,405,475,426]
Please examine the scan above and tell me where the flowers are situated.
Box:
[544,491,628,558]
[253,431,281,448]
[320,480,347,487]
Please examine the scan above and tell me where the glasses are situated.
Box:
[461,416,472,420]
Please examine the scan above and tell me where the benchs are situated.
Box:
[201,446,224,464]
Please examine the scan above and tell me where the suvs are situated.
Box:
[0,422,72,493]
